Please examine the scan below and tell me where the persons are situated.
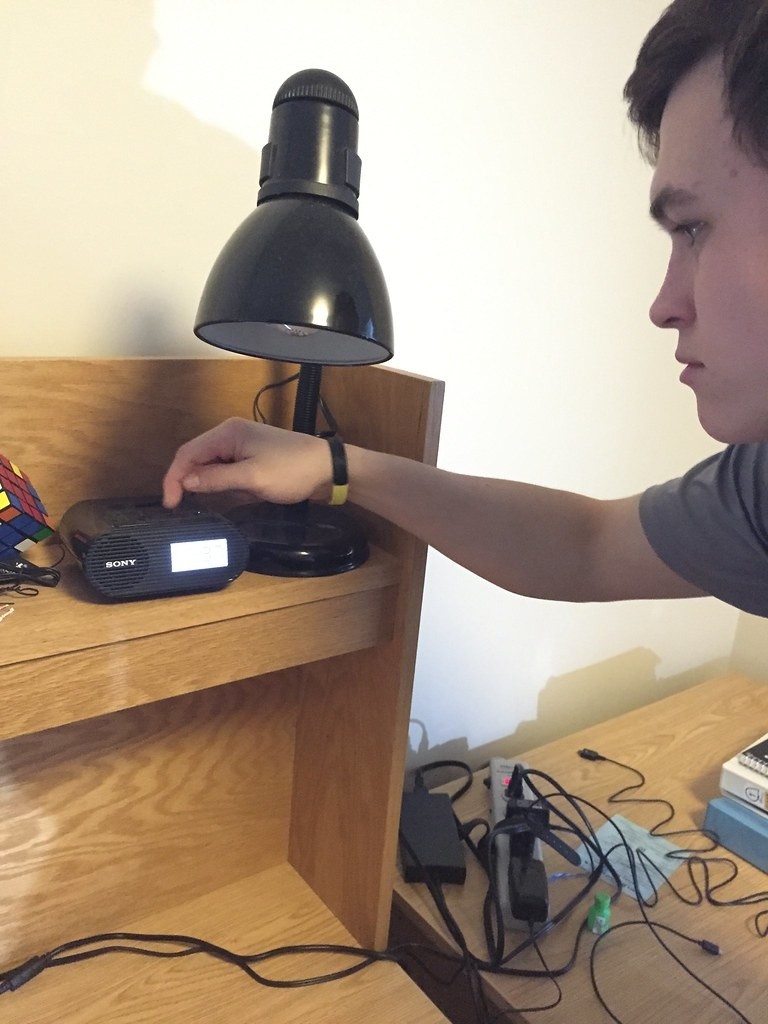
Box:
[163,0,768,617]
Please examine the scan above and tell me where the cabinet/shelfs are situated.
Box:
[0,355,446,954]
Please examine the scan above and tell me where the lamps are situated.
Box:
[194,67,395,576]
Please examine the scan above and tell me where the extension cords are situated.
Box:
[487,757,550,934]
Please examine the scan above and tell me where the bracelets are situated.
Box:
[322,431,348,505]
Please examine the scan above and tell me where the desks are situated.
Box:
[394,671,768,1024]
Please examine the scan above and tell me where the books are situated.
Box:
[703,734,768,871]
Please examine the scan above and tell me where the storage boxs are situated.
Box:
[720,732,768,819]
[703,797,768,874]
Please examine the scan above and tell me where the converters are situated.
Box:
[398,791,467,885]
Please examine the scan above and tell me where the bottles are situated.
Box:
[588,893,612,935]
